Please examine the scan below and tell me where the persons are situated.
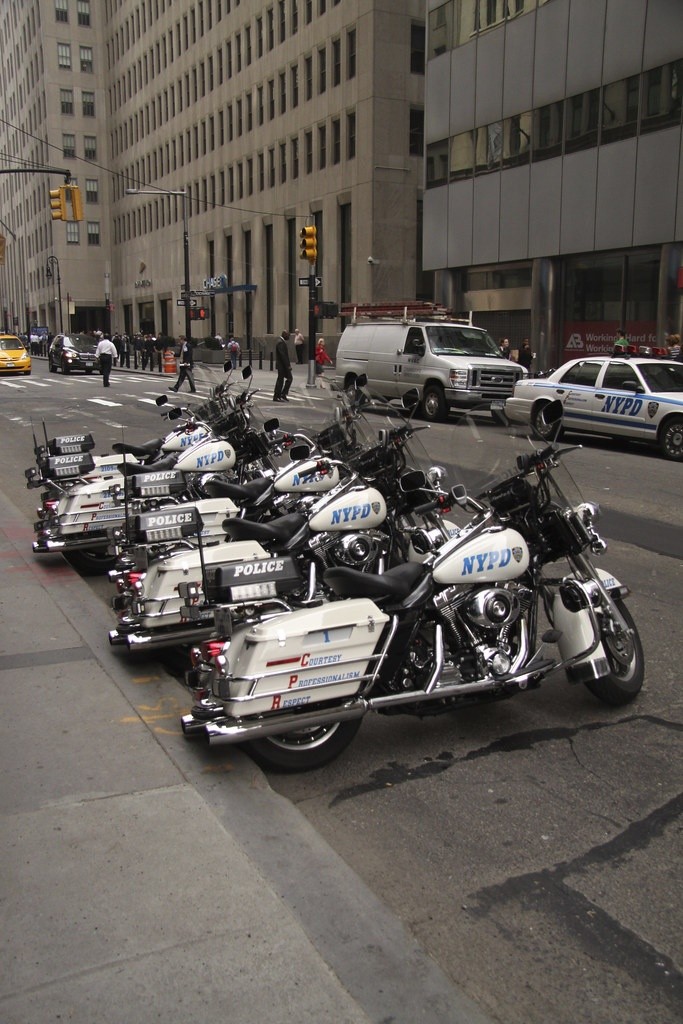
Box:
[614,328,630,348]
[498,337,534,379]
[215,332,224,350]
[227,338,240,369]
[273,330,293,402]
[95,333,119,388]
[294,329,305,364]
[6,331,55,357]
[80,327,164,367]
[315,338,333,389]
[168,335,197,392]
[666,334,681,361]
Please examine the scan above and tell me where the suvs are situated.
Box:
[336,308,527,423]
[50,333,104,375]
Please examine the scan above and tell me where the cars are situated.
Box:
[0,334,32,376]
[503,346,683,462]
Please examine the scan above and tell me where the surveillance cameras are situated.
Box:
[367,256,373,263]
[54,297,58,301]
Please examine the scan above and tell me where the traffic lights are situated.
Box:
[50,190,64,221]
[189,307,209,320]
[314,301,339,319]
[299,225,316,261]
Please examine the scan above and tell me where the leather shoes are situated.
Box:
[281,394,289,401]
[273,396,283,402]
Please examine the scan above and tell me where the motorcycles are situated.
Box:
[181,397,645,785]
[24,360,451,660]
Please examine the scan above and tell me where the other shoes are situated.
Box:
[168,386,177,392]
[188,389,196,393]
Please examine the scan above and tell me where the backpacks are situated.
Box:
[230,341,237,352]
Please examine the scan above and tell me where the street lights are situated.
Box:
[46,255,66,334]
[126,187,192,341]
